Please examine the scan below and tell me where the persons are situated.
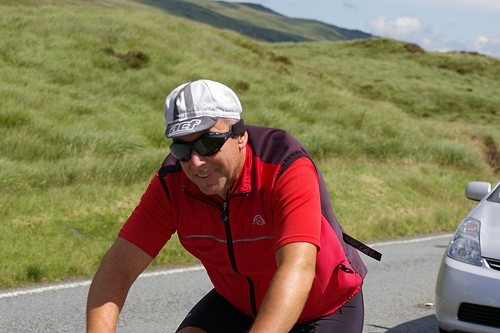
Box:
[86,79,383,333]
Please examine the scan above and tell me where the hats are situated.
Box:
[164,79,242,137]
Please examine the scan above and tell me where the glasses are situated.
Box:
[172,132,223,158]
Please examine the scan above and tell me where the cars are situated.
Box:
[433,180,500,333]
